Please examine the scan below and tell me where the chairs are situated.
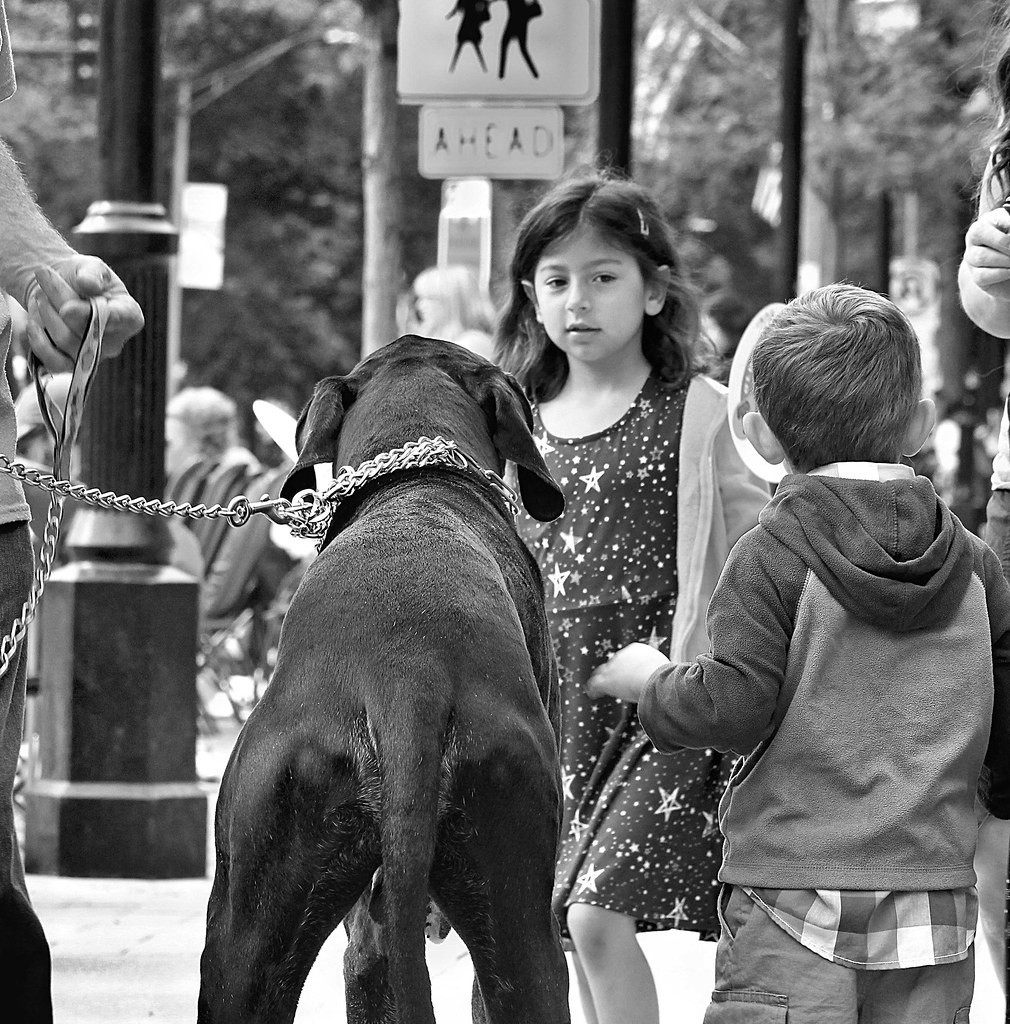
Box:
[161,449,313,739]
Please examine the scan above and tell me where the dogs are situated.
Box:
[197,335,572,1024]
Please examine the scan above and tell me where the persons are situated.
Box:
[959,2,1010,1024]
[588,283,1009,1024]
[0,1,144,1024]
[491,170,774,1024]
[12,264,976,745]
